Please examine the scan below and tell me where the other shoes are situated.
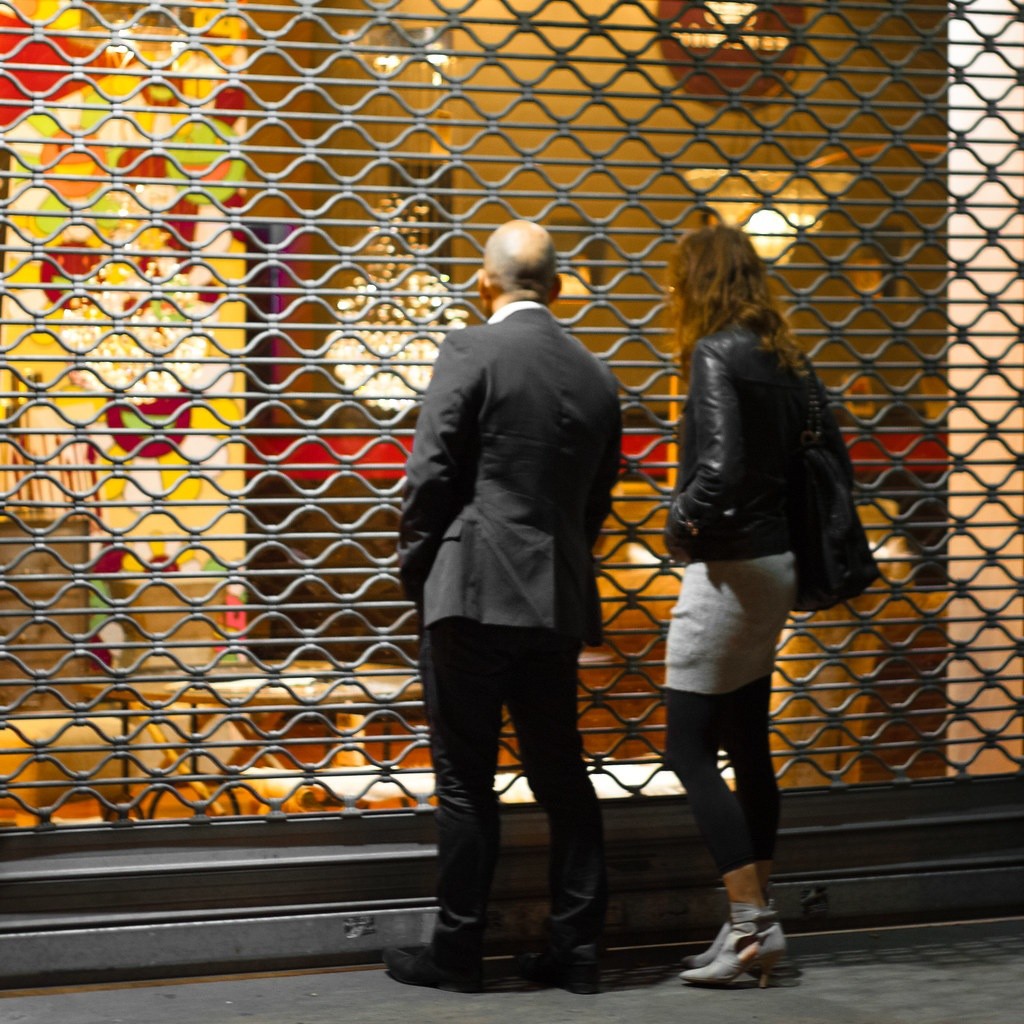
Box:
[383,947,483,992]
[514,949,599,994]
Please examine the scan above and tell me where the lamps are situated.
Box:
[325,189,471,413]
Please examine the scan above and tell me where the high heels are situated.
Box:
[679,899,785,988]
[681,887,773,969]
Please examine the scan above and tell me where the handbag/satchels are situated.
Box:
[791,361,879,611]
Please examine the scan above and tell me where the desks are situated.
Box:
[81,657,425,817]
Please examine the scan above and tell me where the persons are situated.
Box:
[384,220,623,995]
[662,226,808,989]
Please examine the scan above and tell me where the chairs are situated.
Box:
[0,711,168,826]
